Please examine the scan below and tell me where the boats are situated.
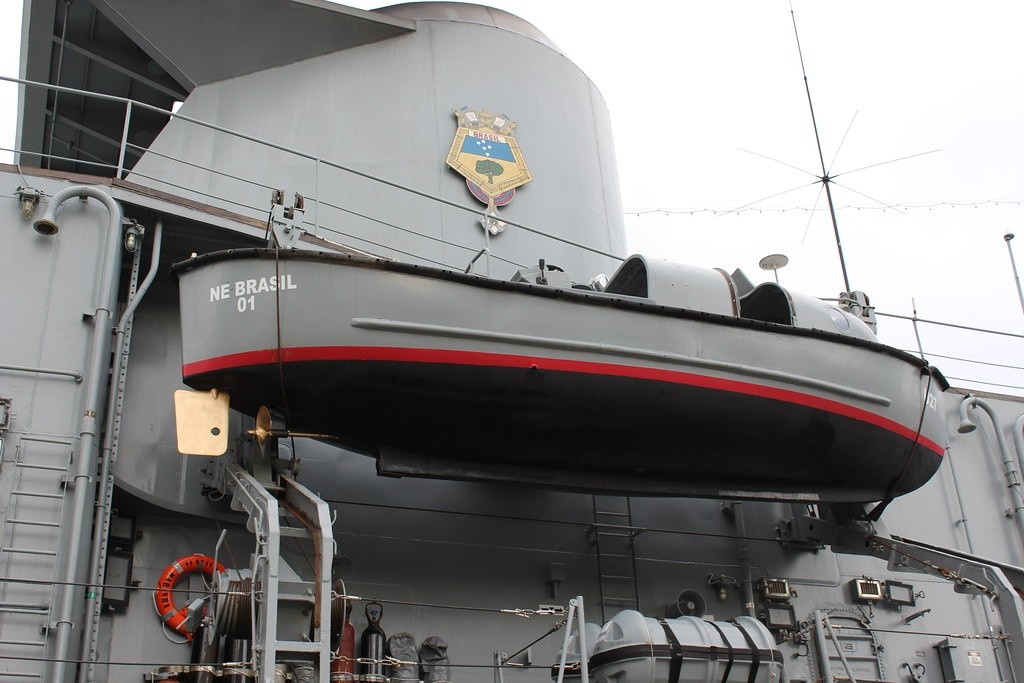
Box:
[169,241,947,531]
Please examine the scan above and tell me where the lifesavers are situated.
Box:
[156,554,226,637]
